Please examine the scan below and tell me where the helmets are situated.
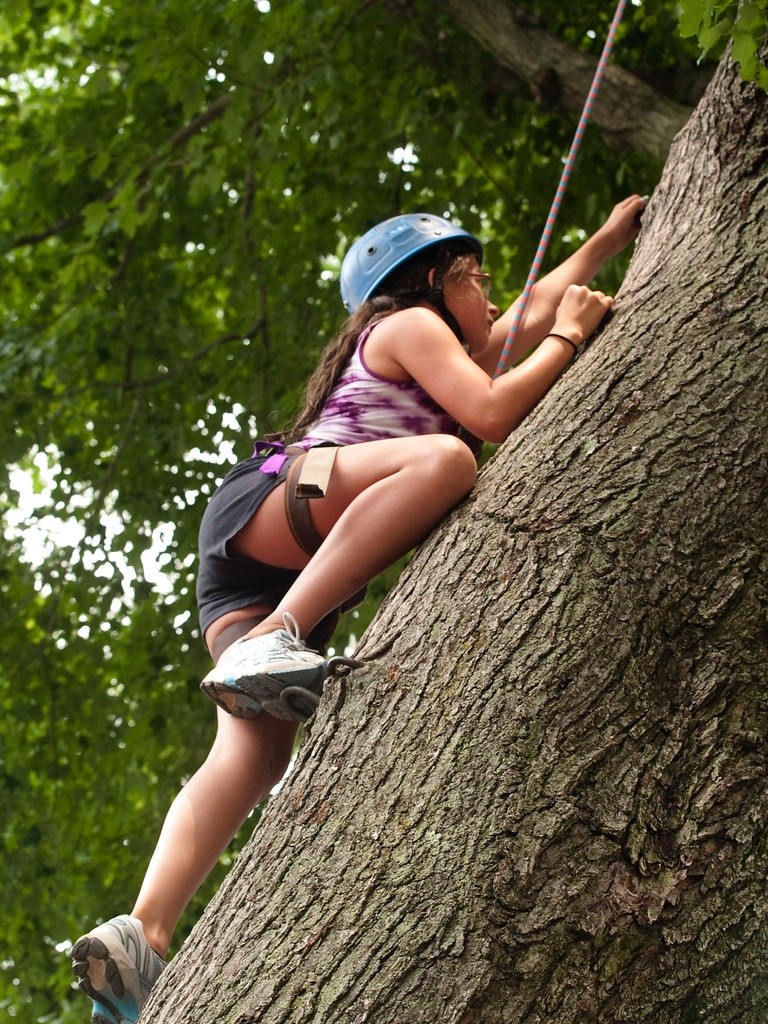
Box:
[340,213,483,318]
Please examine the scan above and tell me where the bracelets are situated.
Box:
[547,333,578,356]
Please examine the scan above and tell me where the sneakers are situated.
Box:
[199,612,327,722]
[70,914,168,1024]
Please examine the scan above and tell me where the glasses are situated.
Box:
[466,273,492,299]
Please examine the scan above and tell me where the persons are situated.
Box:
[71,195,650,1024]
